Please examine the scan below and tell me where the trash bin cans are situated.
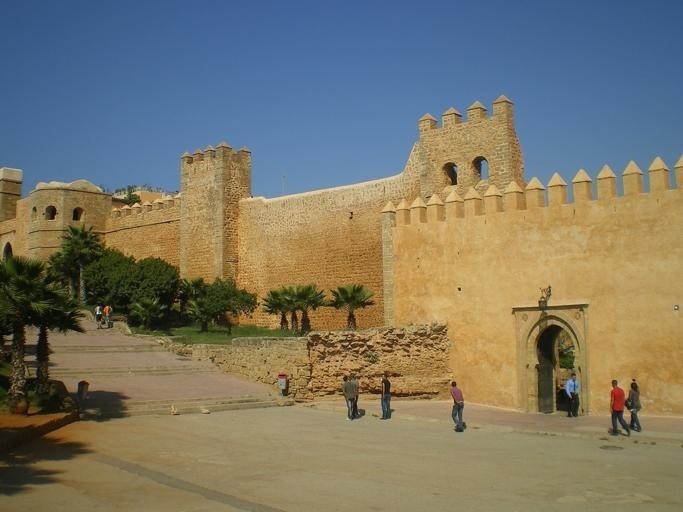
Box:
[108,321,113,329]
[77,381,89,401]
[278,373,288,397]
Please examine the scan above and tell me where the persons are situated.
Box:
[566,372,580,416]
[610,380,631,436]
[349,373,359,418]
[628,383,641,432]
[343,375,356,420]
[94,303,103,329]
[103,304,112,328]
[450,381,466,432]
[381,374,391,419]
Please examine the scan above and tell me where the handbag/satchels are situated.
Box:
[624,391,634,410]
